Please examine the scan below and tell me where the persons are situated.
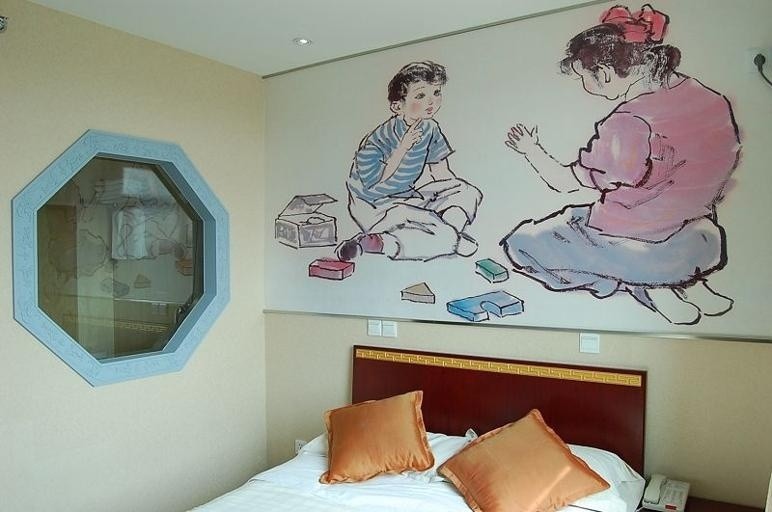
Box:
[499,4,741,326]
[42,177,113,289]
[108,162,188,265]
[335,59,483,262]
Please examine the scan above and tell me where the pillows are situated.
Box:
[321,390,610,510]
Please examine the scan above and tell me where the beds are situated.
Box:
[181,344,647,511]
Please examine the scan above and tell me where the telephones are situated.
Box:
[641,473,691,511]
